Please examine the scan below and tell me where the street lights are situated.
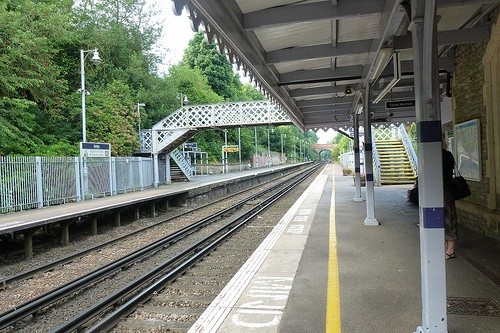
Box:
[76,46,102,201]
[281,133,287,165]
[180,90,190,152]
[266,128,274,168]
[299,138,310,162]
[137,102,146,157]
[293,135,299,162]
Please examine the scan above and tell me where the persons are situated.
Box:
[443,141,458,261]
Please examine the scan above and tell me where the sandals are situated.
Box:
[446,252,455,259]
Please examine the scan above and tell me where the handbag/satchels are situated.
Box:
[452,164,472,199]
